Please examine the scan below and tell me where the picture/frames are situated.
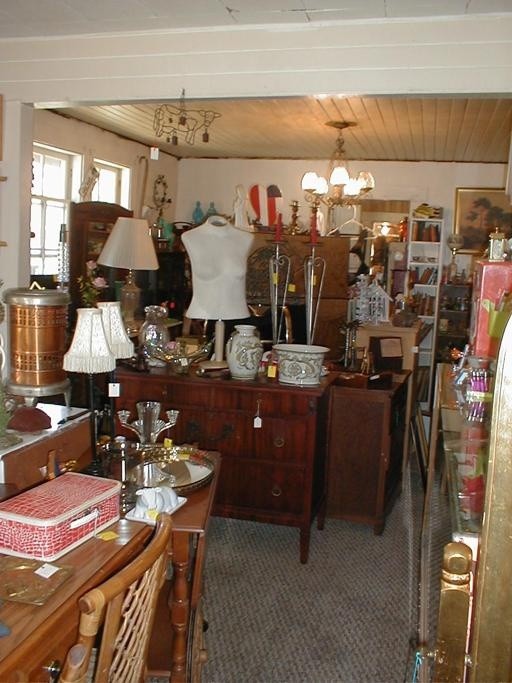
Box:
[454,184,511,252]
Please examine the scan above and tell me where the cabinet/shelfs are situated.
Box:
[439,274,471,368]
[408,216,440,417]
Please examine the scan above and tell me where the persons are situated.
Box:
[181,213,255,319]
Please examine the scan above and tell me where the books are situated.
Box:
[411,218,441,316]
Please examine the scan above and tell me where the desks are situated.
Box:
[351,313,429,367]
[436,360,480,490]
[0,516,160,683]
[314,364,415,537]
[73,438,227,679]
[2,397,91,500]
[98,349,341,566]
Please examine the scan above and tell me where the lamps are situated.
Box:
[95,300,137,455]
[94,211,161,312]
[153,80,227,150]
[61,309,118,456]
[299,118,375,239]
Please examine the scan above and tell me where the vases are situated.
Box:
[276,330,331,397]
[142,299,178,367]
[220,321,263,388]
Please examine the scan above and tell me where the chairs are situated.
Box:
[60,513,186,683]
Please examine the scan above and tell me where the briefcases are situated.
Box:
[0,472,121,563]
